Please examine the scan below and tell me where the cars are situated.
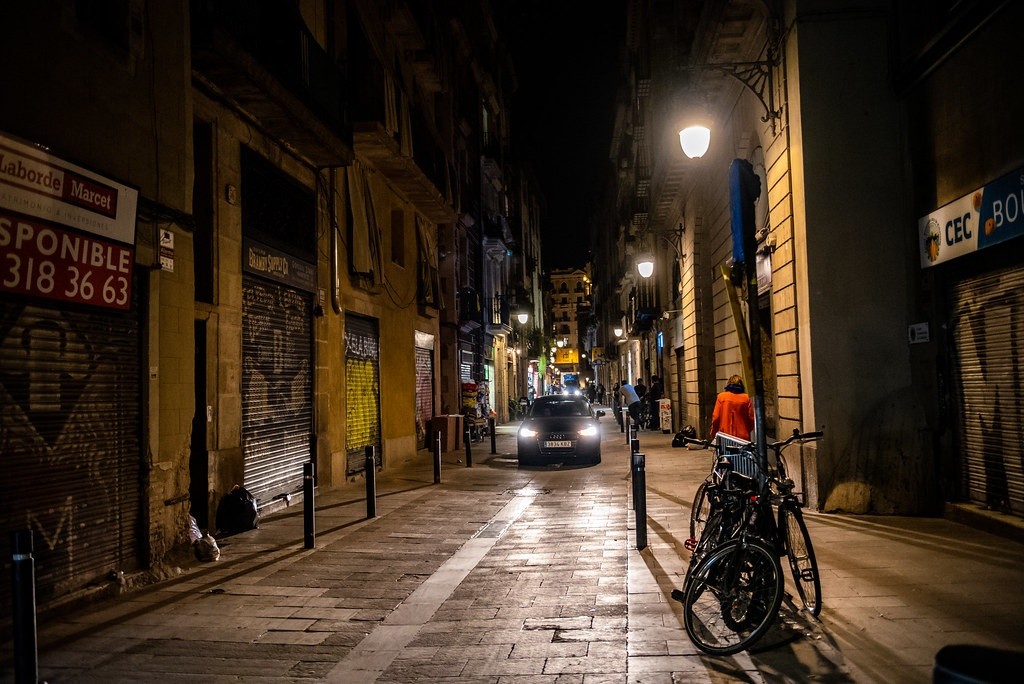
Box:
[516,394,606,466]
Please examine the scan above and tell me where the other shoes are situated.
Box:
[641,422,644,430]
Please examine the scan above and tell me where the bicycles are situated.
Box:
[670,427,822,656]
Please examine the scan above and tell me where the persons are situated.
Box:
[588,383,606,405]
[614,375,662,431]
[709,374,754,440]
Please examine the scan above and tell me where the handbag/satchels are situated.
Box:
[217,488,258,533]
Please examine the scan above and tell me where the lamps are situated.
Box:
[674,59,781,159]
[637,223,684,278]
[611,312,629,337]
[496,292,528,324]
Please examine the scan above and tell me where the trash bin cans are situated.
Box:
[431,414,463,453]
